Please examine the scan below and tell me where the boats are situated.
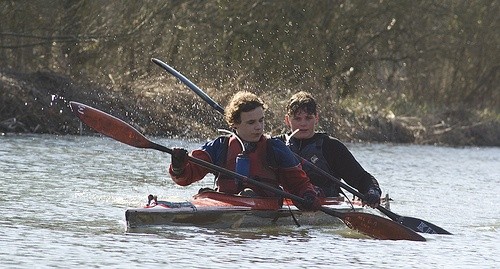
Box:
[124,187,392,232]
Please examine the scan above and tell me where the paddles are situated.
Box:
[66,101,426,243]
[150,56,454,237]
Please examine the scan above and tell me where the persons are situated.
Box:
[262,91,382,209]
[169,92,321,212]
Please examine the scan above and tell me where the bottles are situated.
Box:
[235,153,249,186]
